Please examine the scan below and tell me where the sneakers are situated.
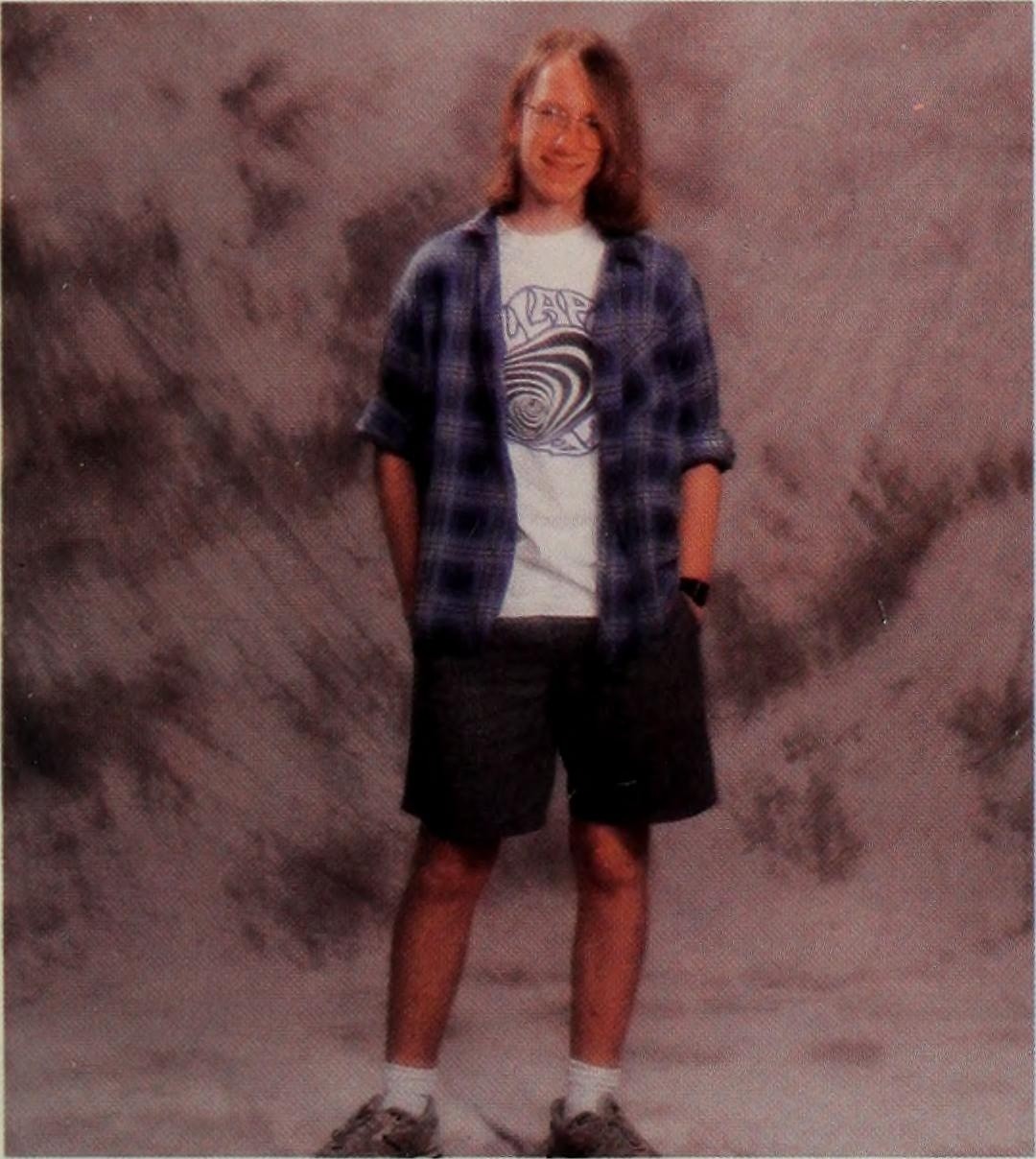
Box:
[543,1092,664,1158]
[313,1093,445,1158]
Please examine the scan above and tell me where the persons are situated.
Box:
[312,33,736,1159]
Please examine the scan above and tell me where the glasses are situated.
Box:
[516,94,606,139]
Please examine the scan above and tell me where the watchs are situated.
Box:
[679,576,711,608]
